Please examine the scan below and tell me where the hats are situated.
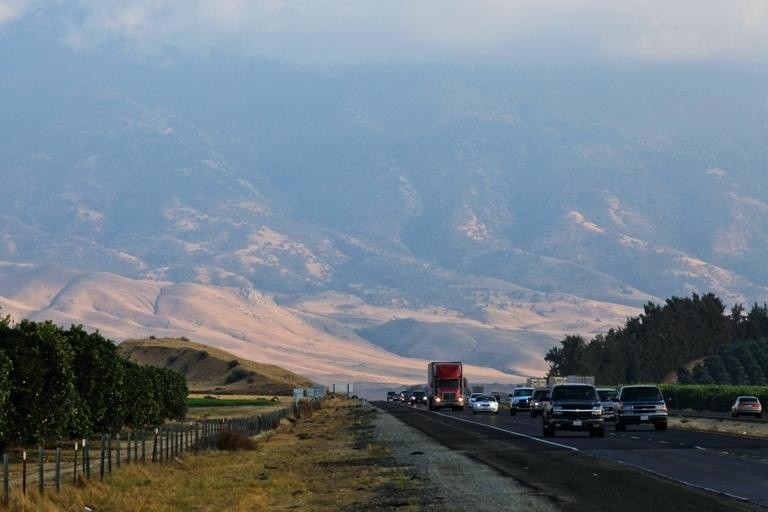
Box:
[383,355,670,443]
[203,395,217,399]
[730,396,762,422]
